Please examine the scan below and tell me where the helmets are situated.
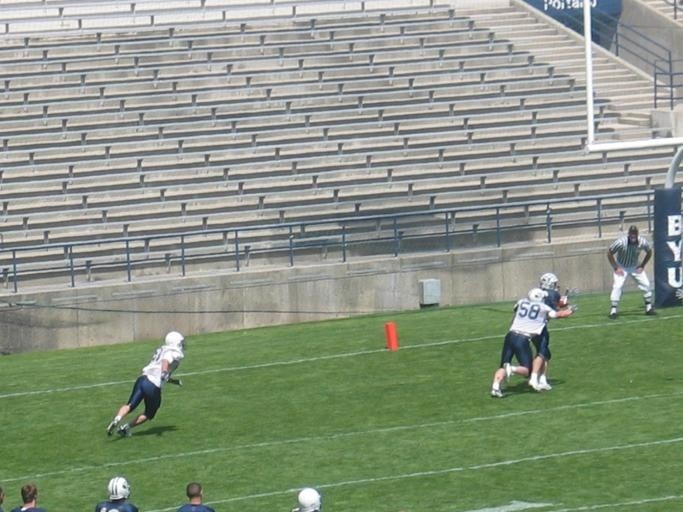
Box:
[107,476,130,500]
[298,488,321,507]
[539,273,560,291]
[629,225,638,234]
[528,288,545,301]
[165,331,184,345]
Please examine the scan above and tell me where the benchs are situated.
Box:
[0,0,683,281]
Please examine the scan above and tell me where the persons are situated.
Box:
[286,485,323,512]
[607,226,658,316]
[94,474,141,512]
[529,273,572,391]
[11,483,48,512]
[103,330,186,436]
[177,482,213,511]
[490,288,579,396]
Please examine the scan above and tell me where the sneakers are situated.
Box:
[107,420,116,435]
[503,362,512,383]
[608,312,616,318]
[528,379,552,392]
[120,425,131,436]
[490,388,503,397]
[646,309,656,315]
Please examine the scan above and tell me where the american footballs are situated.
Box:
[559,295,568,308]
